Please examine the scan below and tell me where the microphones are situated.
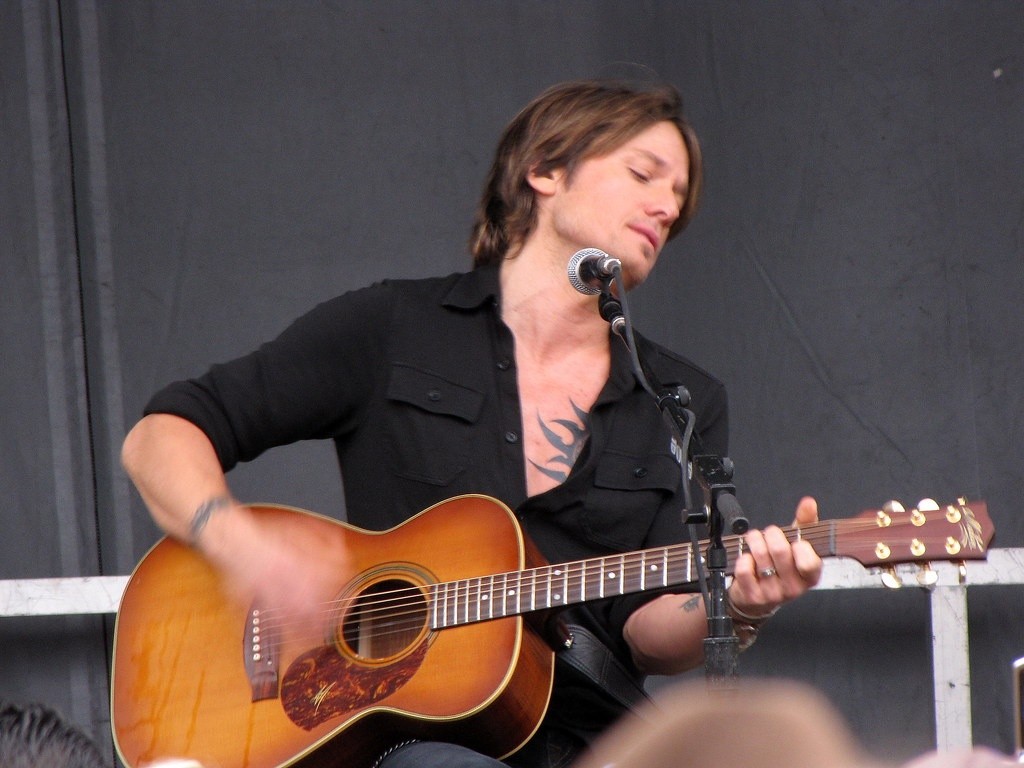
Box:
[566,248,621,296]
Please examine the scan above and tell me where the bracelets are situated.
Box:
[724,594,780,625]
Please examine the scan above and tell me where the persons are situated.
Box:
[119,78,822,768]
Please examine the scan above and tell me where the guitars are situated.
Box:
[110,490,1000,768]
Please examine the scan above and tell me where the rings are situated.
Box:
[758,568,776,580]
